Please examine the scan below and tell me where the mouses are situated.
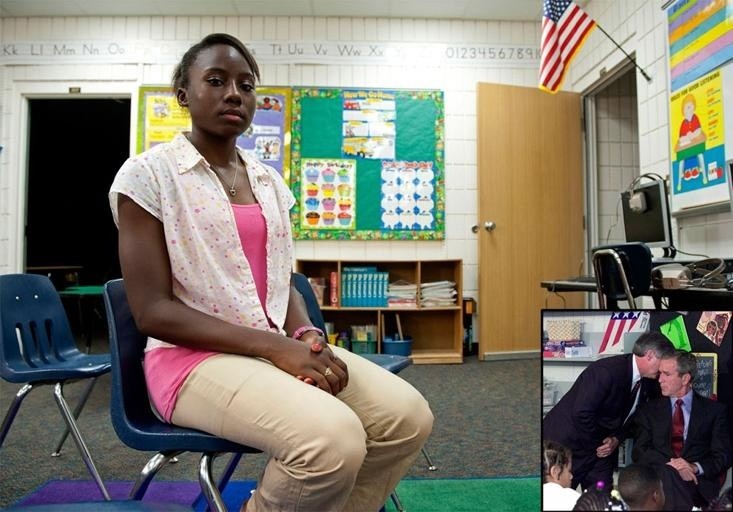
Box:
[727,280,733,291]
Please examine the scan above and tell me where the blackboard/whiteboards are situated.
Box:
[689,352,718,402]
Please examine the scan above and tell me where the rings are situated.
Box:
[324,368,333,376]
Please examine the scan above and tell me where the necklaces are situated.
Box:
[188,136,238,197]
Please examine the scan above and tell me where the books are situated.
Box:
[330,267,459,306]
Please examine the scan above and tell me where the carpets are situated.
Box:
[2,473,542,512]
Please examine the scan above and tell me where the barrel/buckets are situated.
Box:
[383,336,413,356]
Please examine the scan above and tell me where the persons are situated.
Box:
[542,439,666,511]
[632,347,732,512]
[701,321,717,342]
[678,95,700,136]
[110,34,433,511]
[542,333,676,493]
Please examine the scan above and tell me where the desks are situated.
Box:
[539,276,733,311]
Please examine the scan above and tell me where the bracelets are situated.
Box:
[293,326,326,340]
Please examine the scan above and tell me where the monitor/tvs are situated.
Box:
[620,179,676,262]
[727,159,733,202]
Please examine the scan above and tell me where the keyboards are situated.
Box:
[566,276,598,284]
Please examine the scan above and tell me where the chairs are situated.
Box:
[588,240,659,309]
[287,273,438,511]
[1,271,179,504]
[103,275,277,510]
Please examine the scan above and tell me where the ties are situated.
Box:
[672,399,685,458]
[628,379,640,417]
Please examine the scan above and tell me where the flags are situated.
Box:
[598,312,641,355]
[538,0,597,94]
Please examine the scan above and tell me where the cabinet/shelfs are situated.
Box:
[293,256,465,364]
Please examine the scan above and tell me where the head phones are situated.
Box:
[651,256,728,289]
[629,174,670,213]
